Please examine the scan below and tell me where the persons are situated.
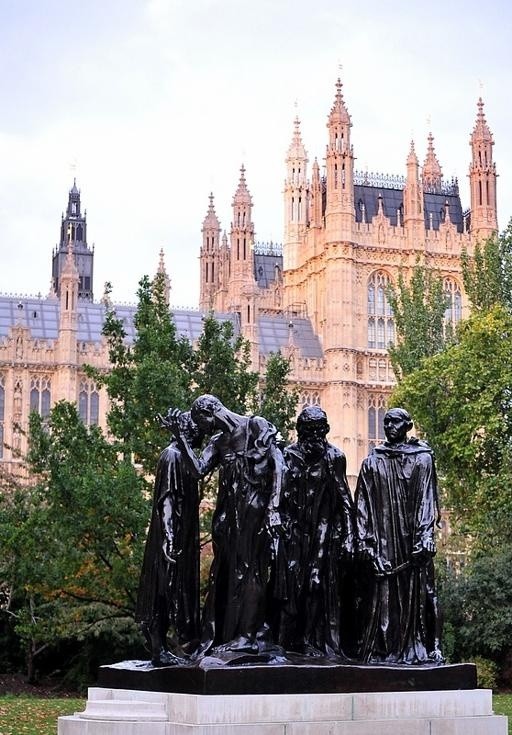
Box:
[158,393,287,654]
[135,411,205,667]
[338,407,446,664]
[267,405,357,659]
